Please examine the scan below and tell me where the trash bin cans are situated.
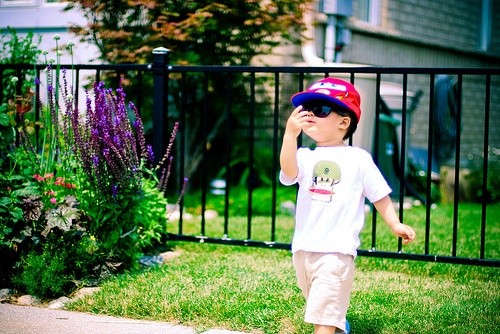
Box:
[376,109,407,198]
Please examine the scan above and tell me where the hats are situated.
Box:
[290,77,361,122]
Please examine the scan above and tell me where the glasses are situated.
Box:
[298,101,344,118]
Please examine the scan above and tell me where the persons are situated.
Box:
[278,77,416,334]
